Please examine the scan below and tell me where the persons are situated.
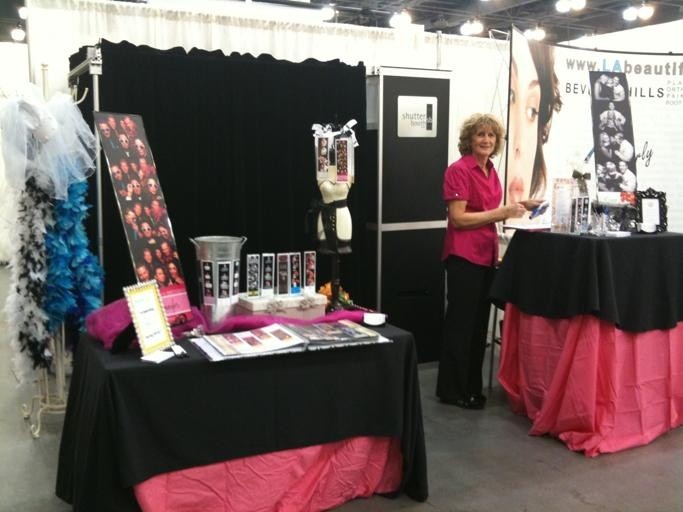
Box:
[435,113,545,409]
[594,74,625,102]
[599,102,627,132]
[506,29,557,204]
[598,131,634,162]
[317,180,353,242]
[97,113,184,288]
[596,161,637,192]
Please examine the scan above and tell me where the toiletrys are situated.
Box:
[529,200,550,220]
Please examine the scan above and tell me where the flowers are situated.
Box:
[319,282,379,313]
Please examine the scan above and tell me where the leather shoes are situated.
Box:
[439,393,486,409]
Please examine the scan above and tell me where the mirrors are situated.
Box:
[633,187,668,235]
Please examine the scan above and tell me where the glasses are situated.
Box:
[132,184,140,188]
[120,139,129,143]
[143,227,151,232]
[135,144,144,149]
[101,128,109,133]
[112,170,121,176]
[148,184,158,189]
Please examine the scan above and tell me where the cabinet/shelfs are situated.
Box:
[365,66,450,363]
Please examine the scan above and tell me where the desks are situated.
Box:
[55,321,428,507]
[488,229,683,458]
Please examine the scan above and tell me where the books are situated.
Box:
[188,317,393,363]
